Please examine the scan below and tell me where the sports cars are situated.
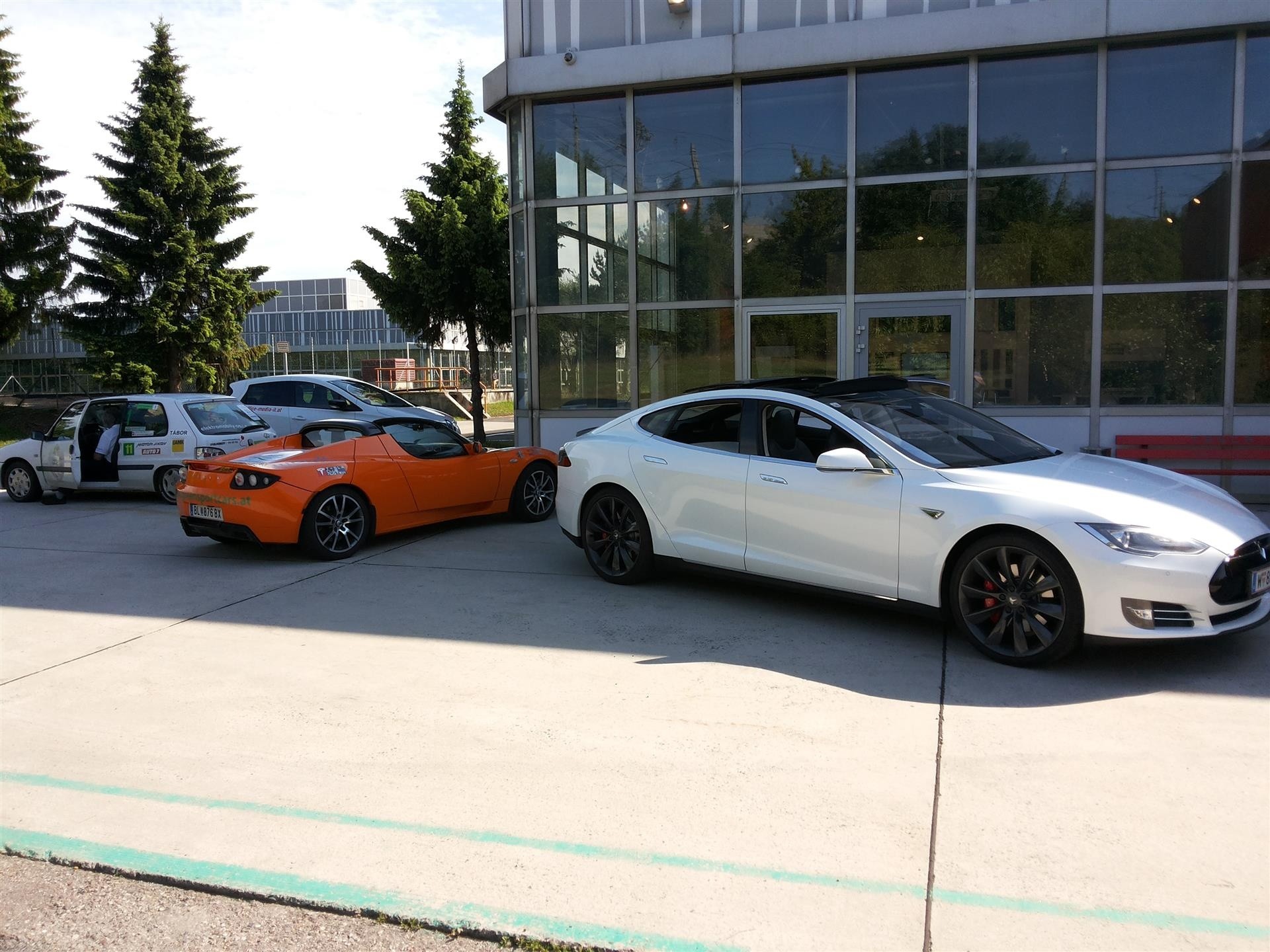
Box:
[176,418,561,557]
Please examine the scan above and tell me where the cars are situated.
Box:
[0,392,279,505]
[555,373,1269,667]
[221,374,461,448]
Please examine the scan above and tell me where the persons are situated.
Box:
[85,407,121,482]
